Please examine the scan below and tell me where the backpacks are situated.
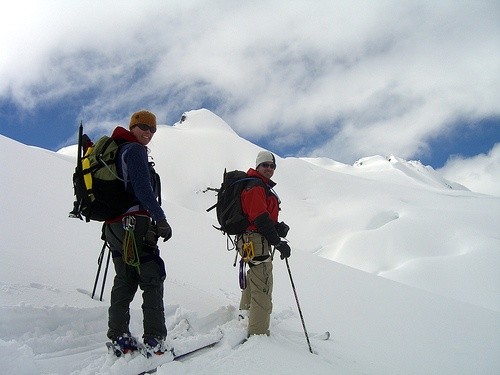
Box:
[216,168,270,235]
[73,135,130,221]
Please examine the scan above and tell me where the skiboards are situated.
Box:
[137,319,224,375]
[231,329,331,351]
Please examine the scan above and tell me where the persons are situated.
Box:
[234,151,291,336]
[102,111,174,356]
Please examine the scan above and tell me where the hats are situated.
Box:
[255,151,275,170]
[129,110,157,130]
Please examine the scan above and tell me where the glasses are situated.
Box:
[136,124,156,133]
[259,162,276,169]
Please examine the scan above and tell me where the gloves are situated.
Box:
[275,240,291,260]
[276,221,290,238]
[156,219,172,242]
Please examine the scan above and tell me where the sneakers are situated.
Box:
[142,340,176,359]
[108,334,144,356]
